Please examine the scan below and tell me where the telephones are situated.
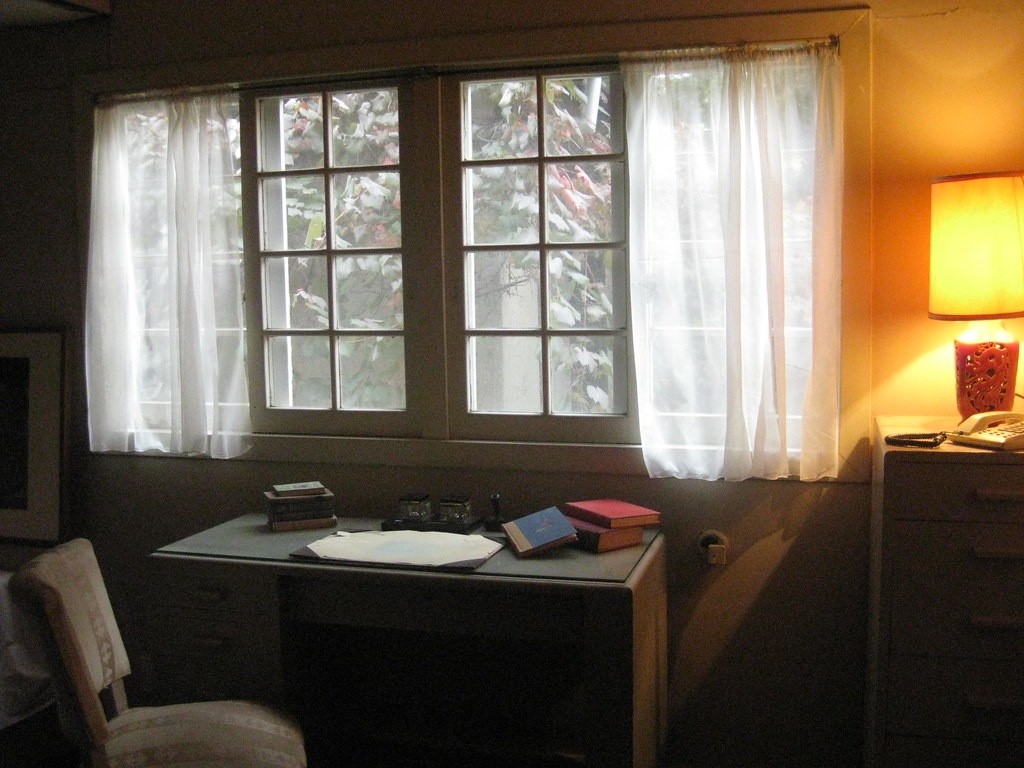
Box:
[945,411,1024,451]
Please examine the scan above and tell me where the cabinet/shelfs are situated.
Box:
[862,443,1024,768]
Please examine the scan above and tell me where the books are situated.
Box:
[498,495,664,558]
[263,482,339,531]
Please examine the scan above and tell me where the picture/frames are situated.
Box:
[0,325,67,547]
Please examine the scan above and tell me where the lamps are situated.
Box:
[928,170,1024,424]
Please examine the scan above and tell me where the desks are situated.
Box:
[147,510,668,768]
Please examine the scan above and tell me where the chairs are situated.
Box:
[8,538,307,768]
